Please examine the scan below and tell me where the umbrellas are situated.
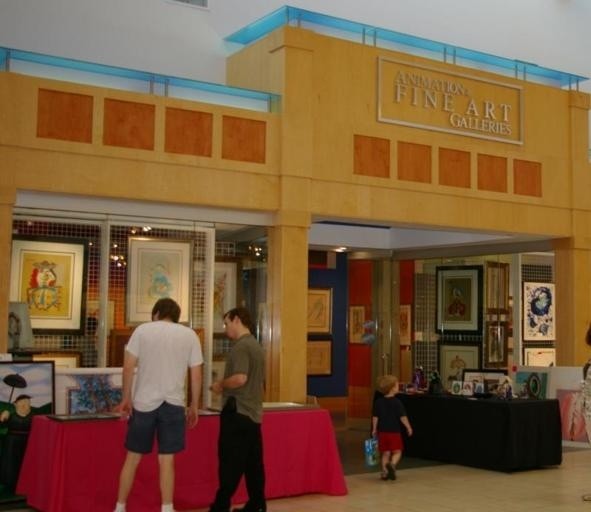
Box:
[3,373,28,422]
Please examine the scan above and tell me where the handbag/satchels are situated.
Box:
[365,437,381,467]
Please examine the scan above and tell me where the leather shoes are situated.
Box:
[232,501,267,512]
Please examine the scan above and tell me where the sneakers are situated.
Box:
[381,462,397,481]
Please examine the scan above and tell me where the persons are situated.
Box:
[111,296,203,512]
[206,307,270,511]
[0,394,38,495]
[370,375,413,482]
[74,316,102,368]
[575,319,591,501]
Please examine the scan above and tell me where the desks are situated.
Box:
[369,388,563,472]
[13,402,347,512]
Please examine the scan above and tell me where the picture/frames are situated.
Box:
[398,303,412,347]
[31,352,79,417]
[307,338,333,377]
[434,259,510,397]
[507,366,552,400]
[46,412,120,422]
[193,257,240,339]
[307,286,333,335]
[0,360,56,434]
[523,281,557,367]
[10,235,89,335]
[206,400,321,410]
[125,234,195,329]
[348,304,368,345]
